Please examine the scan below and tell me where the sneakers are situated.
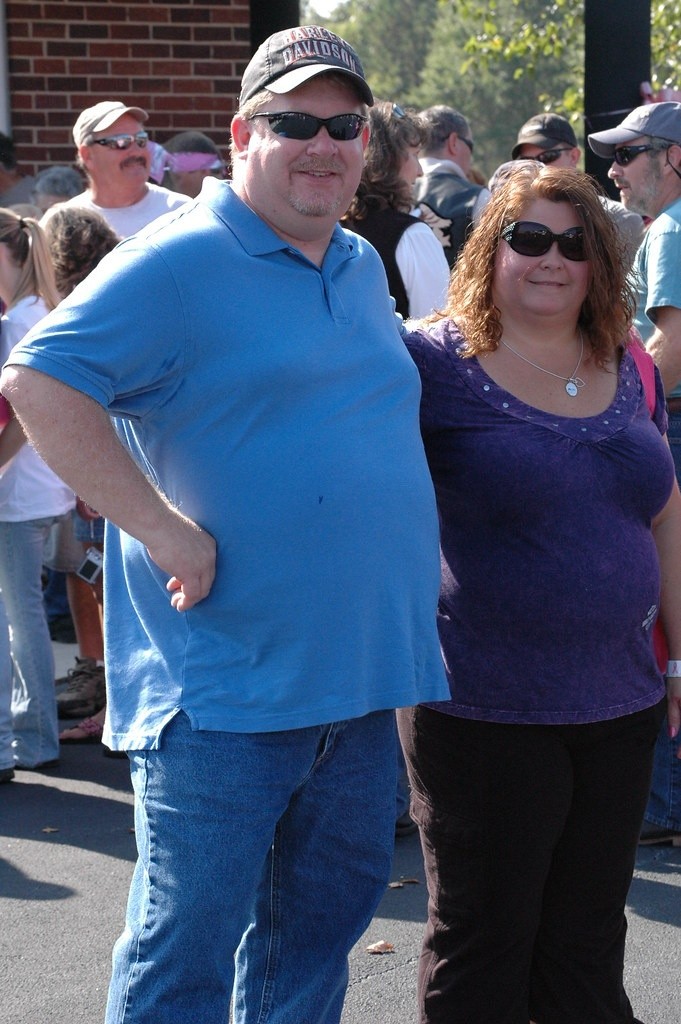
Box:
[55,655,107,720]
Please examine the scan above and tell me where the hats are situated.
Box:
[71,99,149,149]
[510,114,578,159]
[586,101,681,160]
[237,25,375,108]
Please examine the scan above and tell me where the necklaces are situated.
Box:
[493,326,586,397]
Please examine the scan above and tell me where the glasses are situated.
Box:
[388,100,406,121]
[442,134,474,152]
[521,147,576,165]
[93,132,151,150]
[247,109,372,141]
[496,221,592,262]
[612,141,670,168]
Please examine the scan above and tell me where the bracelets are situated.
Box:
[666,659,681,678]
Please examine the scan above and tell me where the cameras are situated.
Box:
[77,547,105,583]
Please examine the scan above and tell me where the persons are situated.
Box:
[0,26,451,1024]
[2,97,681,846]
[397,161,681,1021]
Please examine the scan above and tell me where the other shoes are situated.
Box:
[638,829,681,845]
[56,712,110,744]
